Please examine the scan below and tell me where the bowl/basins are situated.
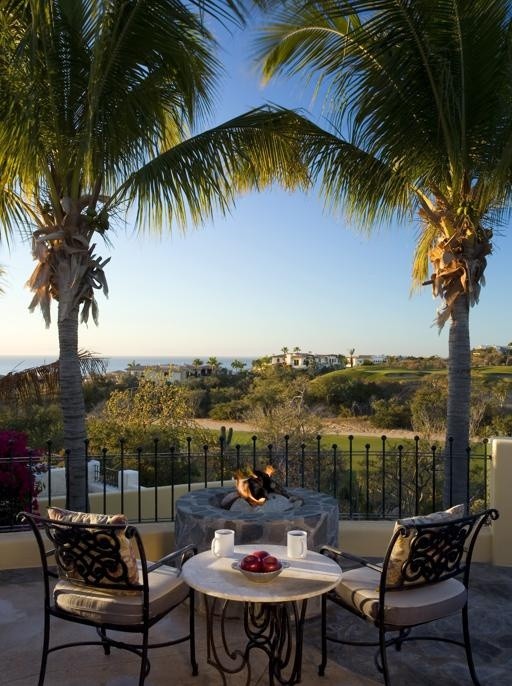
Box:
[231,559,290,583]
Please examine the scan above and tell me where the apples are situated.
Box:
[241,550,282,572]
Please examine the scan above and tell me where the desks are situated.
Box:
[180,543,343,685]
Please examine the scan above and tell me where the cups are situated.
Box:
[287,530,308,559]
[212,529,234,557]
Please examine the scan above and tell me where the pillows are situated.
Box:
[48,506,142,597]
[385,504,464,585]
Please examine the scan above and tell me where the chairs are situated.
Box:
[18,510,200,684]
[314,509,501,685]
